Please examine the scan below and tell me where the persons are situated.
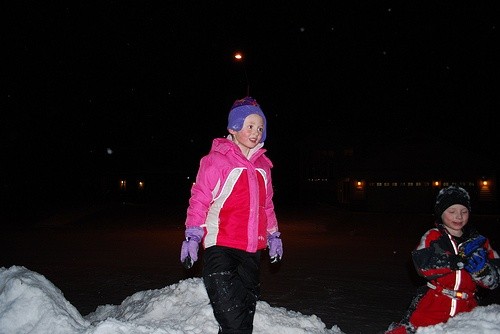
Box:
[181,97,283,334]
[384,186,500,334]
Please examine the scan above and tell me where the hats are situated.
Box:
[436,182,471,215]
[227,96,266,143]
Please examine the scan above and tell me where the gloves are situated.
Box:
[266,232,283,264]
[460,236,488,274]
[180,228,204,268]
